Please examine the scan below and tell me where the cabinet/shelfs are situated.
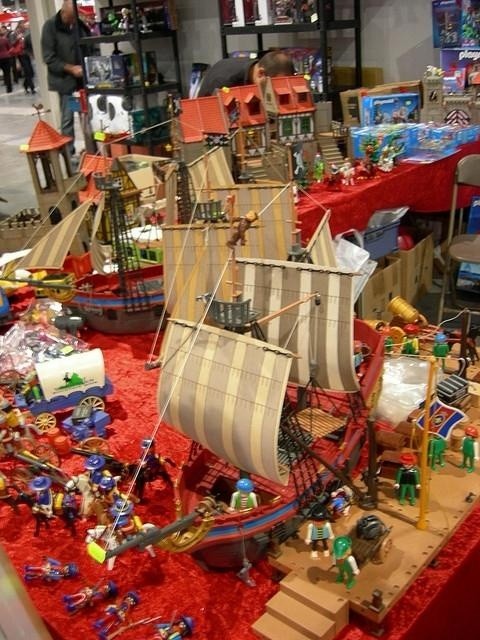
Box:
[219,0,361,121]
[74,0,182,153]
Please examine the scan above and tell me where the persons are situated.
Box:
[2,24,21,84]
[0,27,14,93]
[40,0,97,157]
[9,20,37,95]
[196,51,296,98]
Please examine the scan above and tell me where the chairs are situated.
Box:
[439,155,480,326]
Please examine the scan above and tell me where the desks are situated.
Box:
[0,320,480,640]
[295,141,480,247]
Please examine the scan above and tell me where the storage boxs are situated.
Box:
[400,233,434,306]
[356,257,402,325]
